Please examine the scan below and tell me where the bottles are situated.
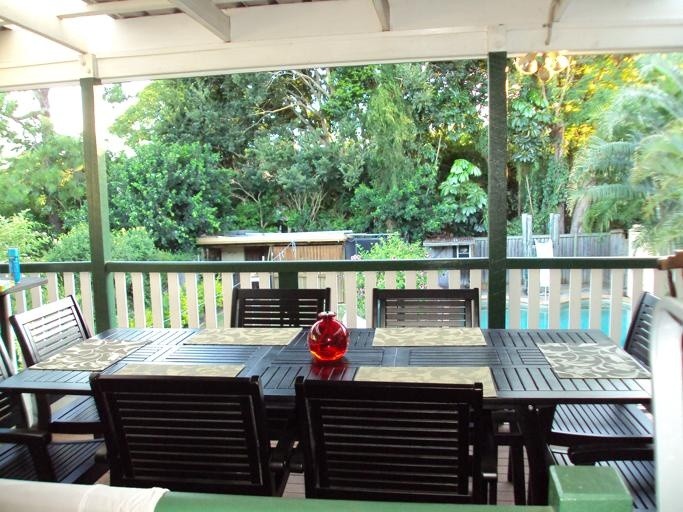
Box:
[310,359,347,382]
[307,313,349,361]
[8,247,20,283]
